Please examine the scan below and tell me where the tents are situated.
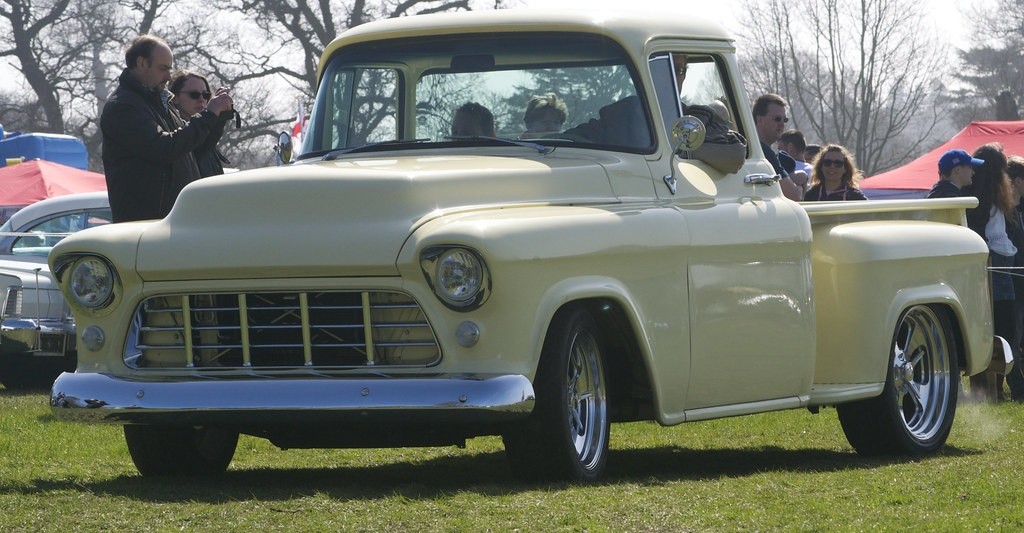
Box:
[0,157,108,206]
[860,121,1024,200]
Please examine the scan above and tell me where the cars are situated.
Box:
[0,190,115,258]
[0,257,78,389]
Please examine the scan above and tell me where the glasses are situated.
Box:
[174,90,211,100]
[675,64,686,74]
[772,117,788,122]
[823,159,844,167]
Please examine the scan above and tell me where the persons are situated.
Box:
[451,56,867,201]
[100,35,234,224]
[927,143,1024,405]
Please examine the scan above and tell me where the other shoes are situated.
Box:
[970,382,1010,403]
[957,388,969,403]
[1011,392,1024,404]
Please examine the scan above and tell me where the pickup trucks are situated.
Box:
[48,0,1014,482]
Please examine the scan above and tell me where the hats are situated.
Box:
[938,150,985,172]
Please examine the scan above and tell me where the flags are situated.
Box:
[289,103,306,139]
